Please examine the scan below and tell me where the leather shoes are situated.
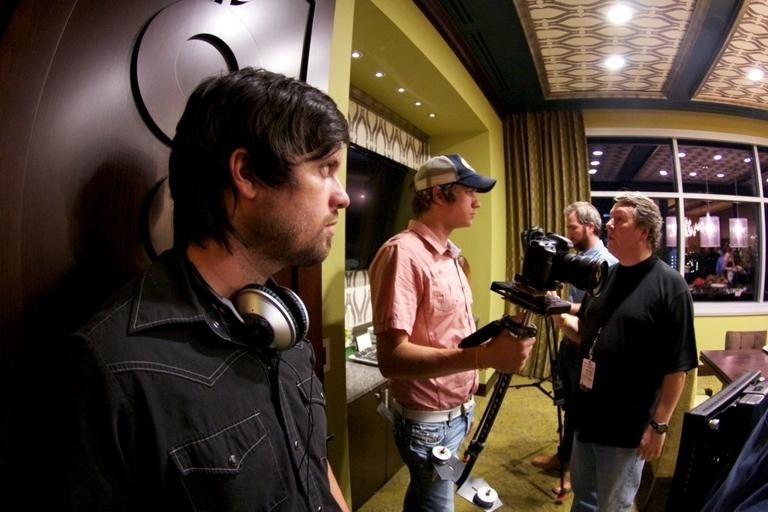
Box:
[532,454,570,468]
[552,478,572,495]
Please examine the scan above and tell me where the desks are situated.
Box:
[699,348,768,387]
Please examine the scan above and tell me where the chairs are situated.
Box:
[722,330,768,390]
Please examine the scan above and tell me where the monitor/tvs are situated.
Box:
[346,144,407,269]
[663,367,768,512]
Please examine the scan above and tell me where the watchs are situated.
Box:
[648,417,668,434]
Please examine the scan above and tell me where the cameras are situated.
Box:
[520,226,611,299]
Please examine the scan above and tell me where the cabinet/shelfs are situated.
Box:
[347,381,406,511]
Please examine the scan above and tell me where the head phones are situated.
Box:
[176,254,311,351]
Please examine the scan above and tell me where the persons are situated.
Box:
[531,199,620,470]
[368,152,537,512]
[684,232,744,286]
[31,66,352,512]
[544,195,697,512]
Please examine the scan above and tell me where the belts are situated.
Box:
[392,394,475,423]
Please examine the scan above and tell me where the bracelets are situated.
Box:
[473,345,488,372]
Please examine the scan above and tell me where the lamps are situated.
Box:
[665,164,749,249]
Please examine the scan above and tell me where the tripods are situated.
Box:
[455,280,570,501]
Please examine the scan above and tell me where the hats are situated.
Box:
[413,153,497,193]
